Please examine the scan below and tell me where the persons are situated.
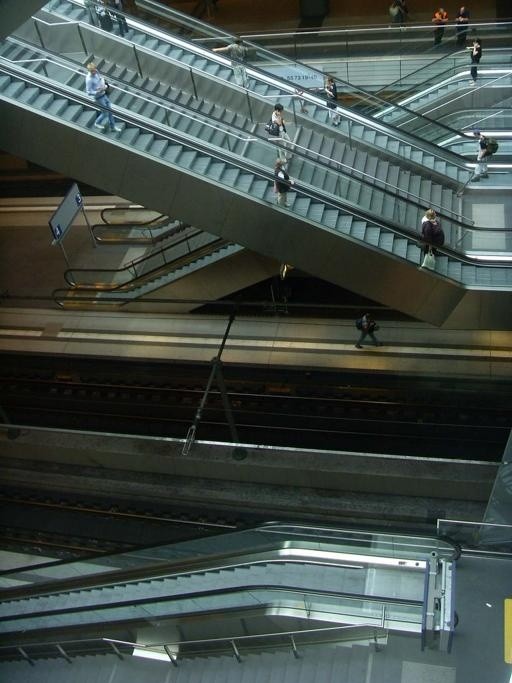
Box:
[85,61,123,132]
[465,37,483,86]
[469,128,490,181]
[388,0,405,23]
[431,7,449,44]
[455,5,470,47]
[425,210,440,232]
[112,0,129,37]
[272,157,295,207]
[212,34,259,89]
[397,0,408,25]
[93,0,114,32]
[354,308,384,349]
[419,208,444,271]
[271,103,293,163]
[325,76,341,126]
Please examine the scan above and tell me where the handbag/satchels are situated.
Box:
[264,120,279,137]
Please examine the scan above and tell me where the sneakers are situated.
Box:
[356,344,362,349]
[110,126,122,132]
[376,344,384,347]
[471,177,480,182]
[481,175,488,178]
[94,123,105,130]
[331,122,341,127]
[285,202,291,207]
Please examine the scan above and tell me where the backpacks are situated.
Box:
[478,135,498,155]
[356,313,366,330]
